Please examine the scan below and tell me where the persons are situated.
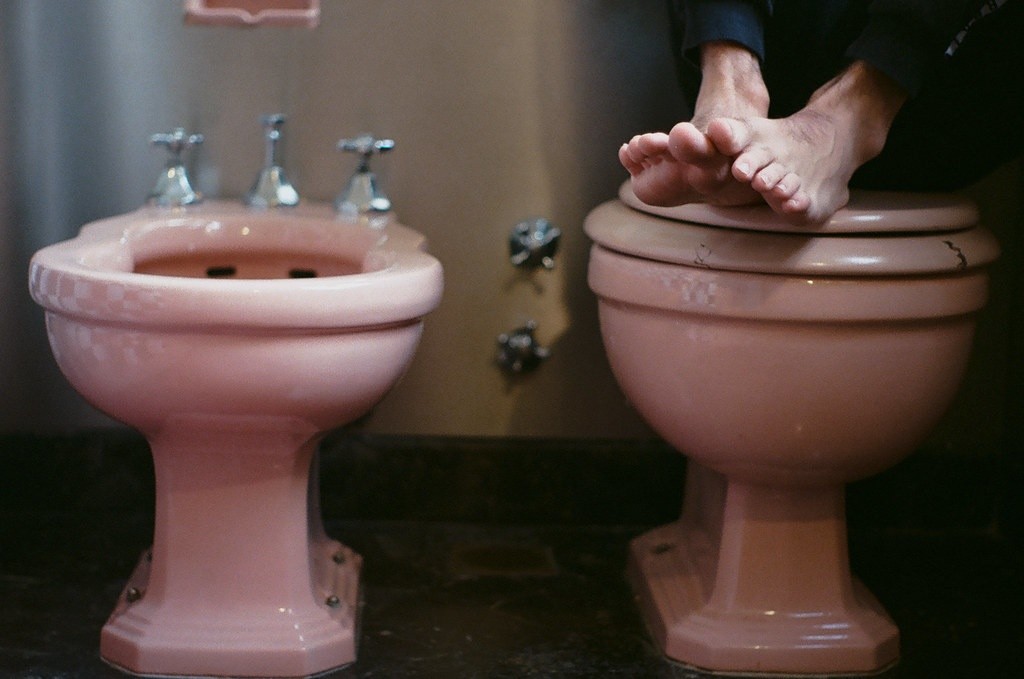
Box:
[618,0,982,229]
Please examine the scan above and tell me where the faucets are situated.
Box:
[250,110,300,211]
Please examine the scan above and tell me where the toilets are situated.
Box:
[583,186,1001,678]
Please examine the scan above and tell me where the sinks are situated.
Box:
[31,202,446,439]
